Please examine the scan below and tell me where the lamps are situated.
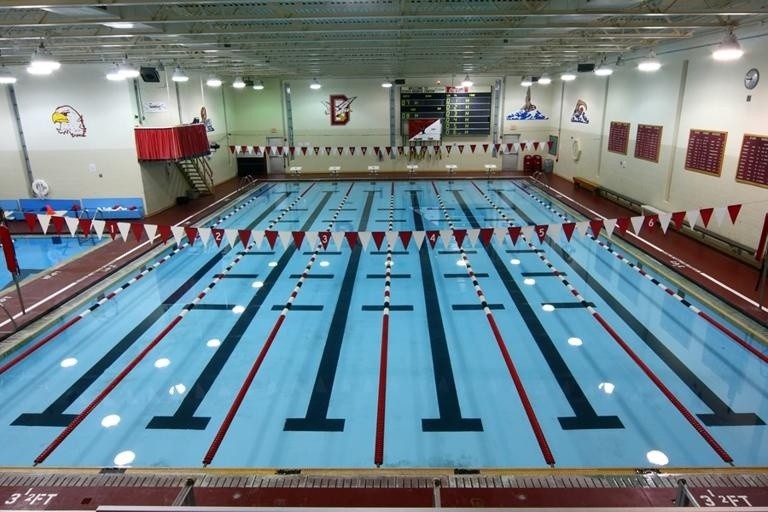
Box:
[560,67,576,81]
[233,76,245,88]
[1,64,15,84]
[171,68,188,82]
[252,79,265,89]
[538,72,551,85]
[310,78,321,88]
[25,43,61,76]
[638,52,660,71]
[521,76,532,86]
[206,73,222,87]
[106,57,141,82]
[460,75,473,87]
[713,34,744,61]
[595,61,612,76]
[381,77,393,89]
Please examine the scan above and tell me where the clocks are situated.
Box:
[744,68,760,89]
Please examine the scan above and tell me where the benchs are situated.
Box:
[0,196,145,223]
[571,176,602,199]
